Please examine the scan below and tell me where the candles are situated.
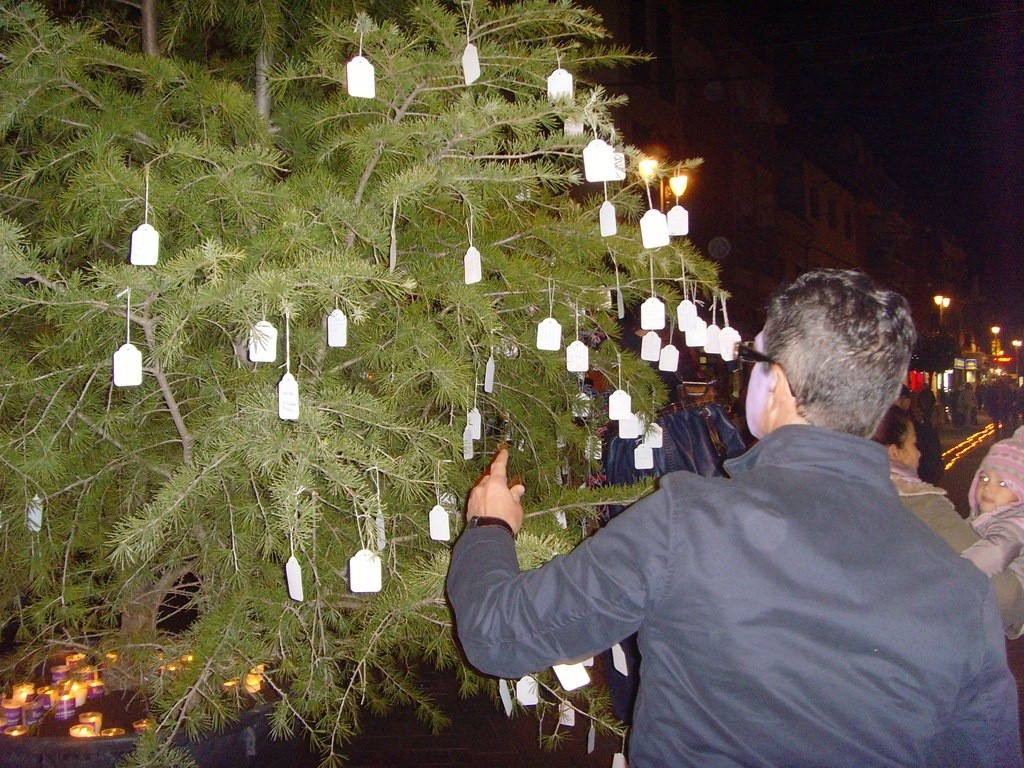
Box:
[0,649,265,738]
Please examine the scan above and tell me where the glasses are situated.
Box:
[734,340,797,397]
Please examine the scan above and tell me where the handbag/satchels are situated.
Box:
[601,397,749,526]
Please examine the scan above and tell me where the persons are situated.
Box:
[445,269,1024,768]
[958,424,1024,594]
[889,376,1024,487]
[867,403,1024,641]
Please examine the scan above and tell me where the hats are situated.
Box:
[968,426,1023,511]
